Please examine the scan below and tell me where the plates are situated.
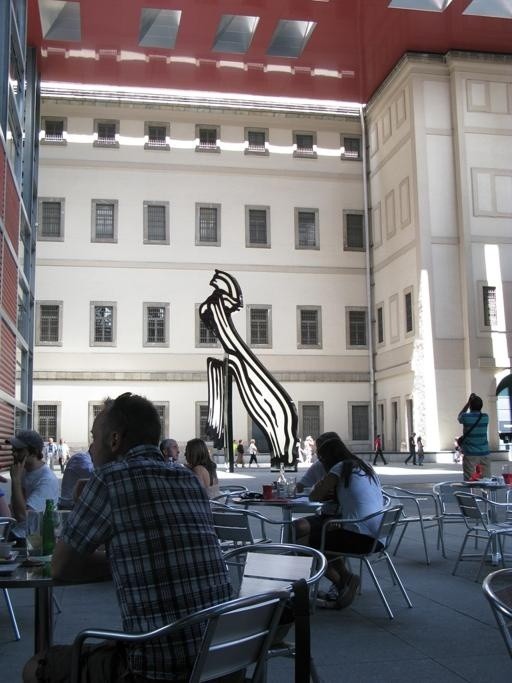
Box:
[0,550,19,563]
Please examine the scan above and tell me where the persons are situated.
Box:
[302,435,315,465]
[373,434,389,465]
[5,430,61,546]
[233,439,258,468]
[60,443,95,508]
[295,437,303,463]
[22,393,231,683]
[290,432,344,548]
[309,438,387,607]
[59,439,71,469]
[406,433,417,465]
[457,393,490,479]
[416,437,424,465]
[159,439,180,461]
[44,438,57,468]
[184,438,218,486]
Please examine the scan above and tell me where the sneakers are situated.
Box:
[338,574,360,608]
[313,585,339,610]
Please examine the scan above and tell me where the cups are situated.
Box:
[53,510,71,547]
[263,485,272,498]
[0,543,11,557]
[287,477,296,498]
[503,473,512,484]
[498,476,503,484]
[26,510,43,561]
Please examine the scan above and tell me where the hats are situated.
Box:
[5,430,44,448]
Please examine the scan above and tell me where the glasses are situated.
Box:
[115,391,131,427]
[10,447,27,451]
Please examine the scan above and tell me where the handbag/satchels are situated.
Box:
[455,437,463,452]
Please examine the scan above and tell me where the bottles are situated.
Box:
[41,499,55,555]
[277,463,288,498]
